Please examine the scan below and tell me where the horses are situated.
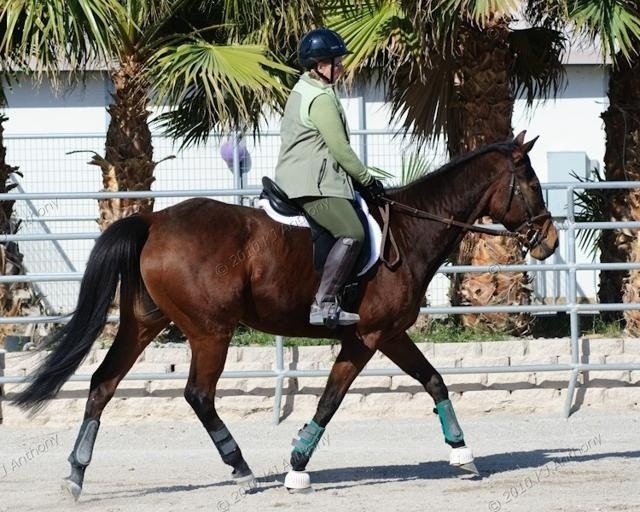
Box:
[5,128,562,503]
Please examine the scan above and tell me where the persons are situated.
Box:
[274,27,386,326]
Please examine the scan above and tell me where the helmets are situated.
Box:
[298,29,354,68]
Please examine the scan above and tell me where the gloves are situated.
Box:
[366,177,385,198]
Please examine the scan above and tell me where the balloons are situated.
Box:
[219,140,245,165]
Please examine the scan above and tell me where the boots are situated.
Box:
[310,235,361,325]
